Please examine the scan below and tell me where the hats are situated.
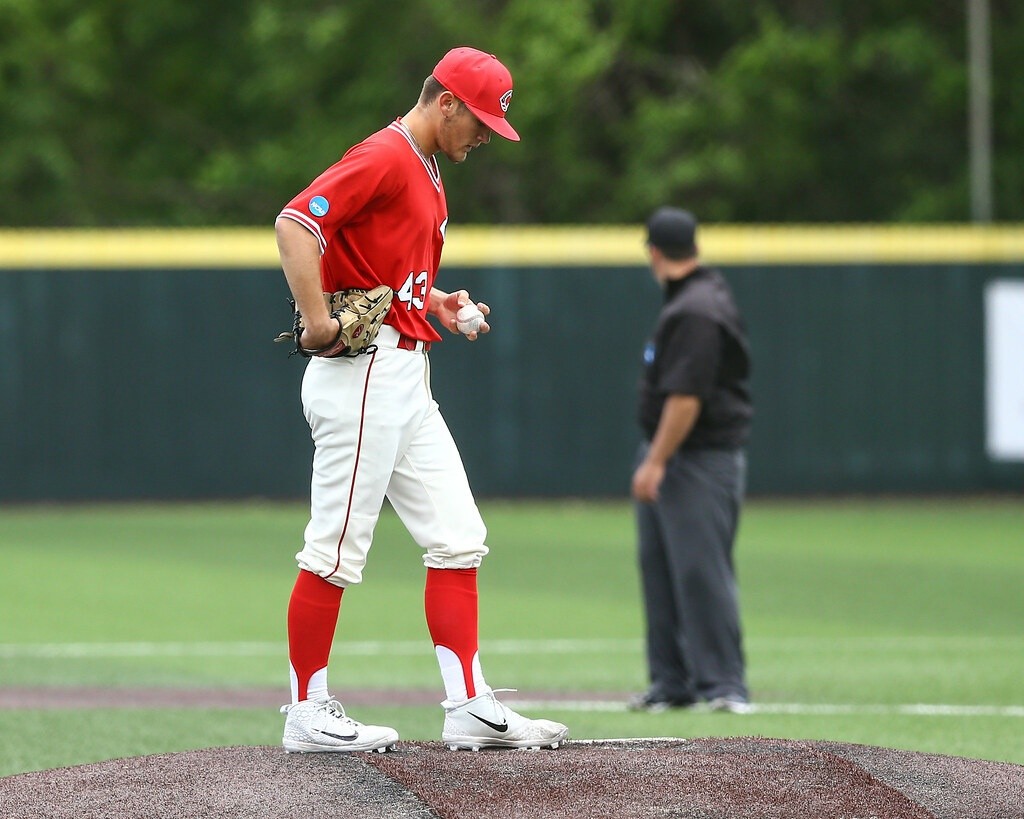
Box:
[432,47,521,142]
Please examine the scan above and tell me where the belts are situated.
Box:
[398,334,431,351]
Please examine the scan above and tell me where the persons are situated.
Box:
[627,204,758,720]
[273,45,567,754]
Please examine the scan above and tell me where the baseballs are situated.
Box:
[456,305,485,334]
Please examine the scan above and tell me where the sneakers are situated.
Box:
[440,692,569,751]
[280,699,398,753]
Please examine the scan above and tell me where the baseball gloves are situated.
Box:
[291,285,393,359]
